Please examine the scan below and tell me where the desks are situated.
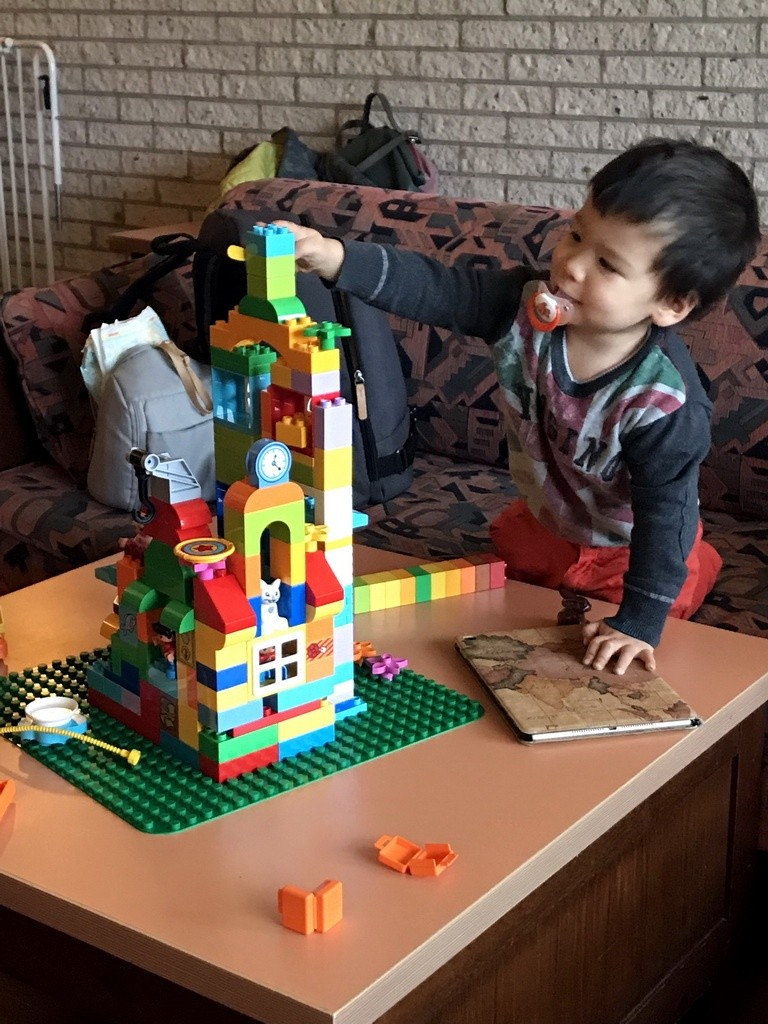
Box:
[0,541,768,1023]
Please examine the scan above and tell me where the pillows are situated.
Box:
[2,254,208,483]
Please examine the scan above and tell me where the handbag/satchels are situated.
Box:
[87,340,218,511]
[330,92,438,193]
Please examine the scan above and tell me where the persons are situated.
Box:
[255,139,759,675]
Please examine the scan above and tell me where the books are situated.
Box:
[452,624,705,745]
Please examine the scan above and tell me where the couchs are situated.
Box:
[1,178,768,635]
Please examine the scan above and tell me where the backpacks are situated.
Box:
[152,208,418,513]
